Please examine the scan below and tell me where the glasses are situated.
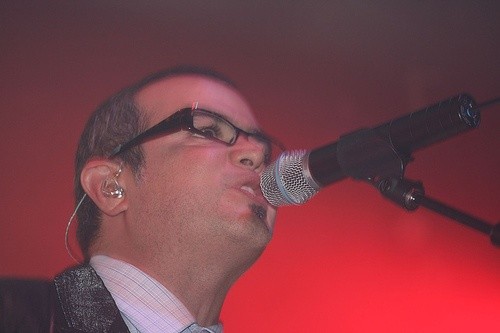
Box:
[108,106,287,165]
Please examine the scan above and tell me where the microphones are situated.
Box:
[260,93,481,207]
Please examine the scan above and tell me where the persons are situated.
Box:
[0,64,278,333]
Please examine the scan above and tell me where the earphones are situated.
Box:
[102,181,124,199]
